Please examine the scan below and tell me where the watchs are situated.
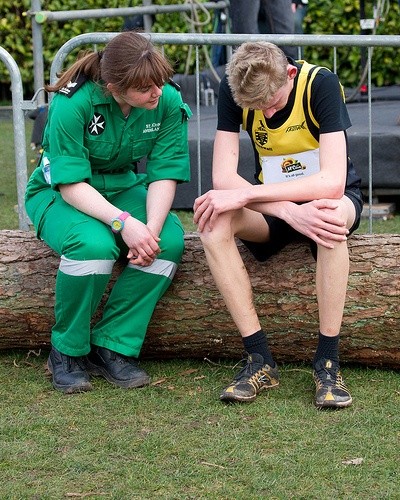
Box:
[111,211,130,234]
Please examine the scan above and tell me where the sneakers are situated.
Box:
[80,341,151,389]
[219,361,280,401]
[47,347,93,392]
[313,359,352,407]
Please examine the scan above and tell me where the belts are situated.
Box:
[92,167,127,175]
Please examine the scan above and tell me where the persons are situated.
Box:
[193,41,364,407]
[25,33,193,393]
[206,0,308,68]
